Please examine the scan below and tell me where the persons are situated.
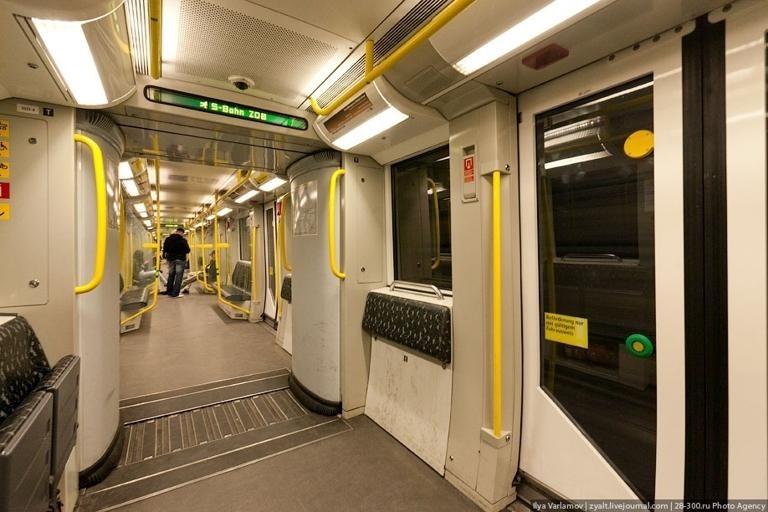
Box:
[162,228,190,297]
[178,249,217,290]
[132,249,167,292]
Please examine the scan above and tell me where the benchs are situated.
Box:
[117,272,150,334]
[214,259,251,321]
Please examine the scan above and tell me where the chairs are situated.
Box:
[0,389,54,512]
[0,311,82,490]
